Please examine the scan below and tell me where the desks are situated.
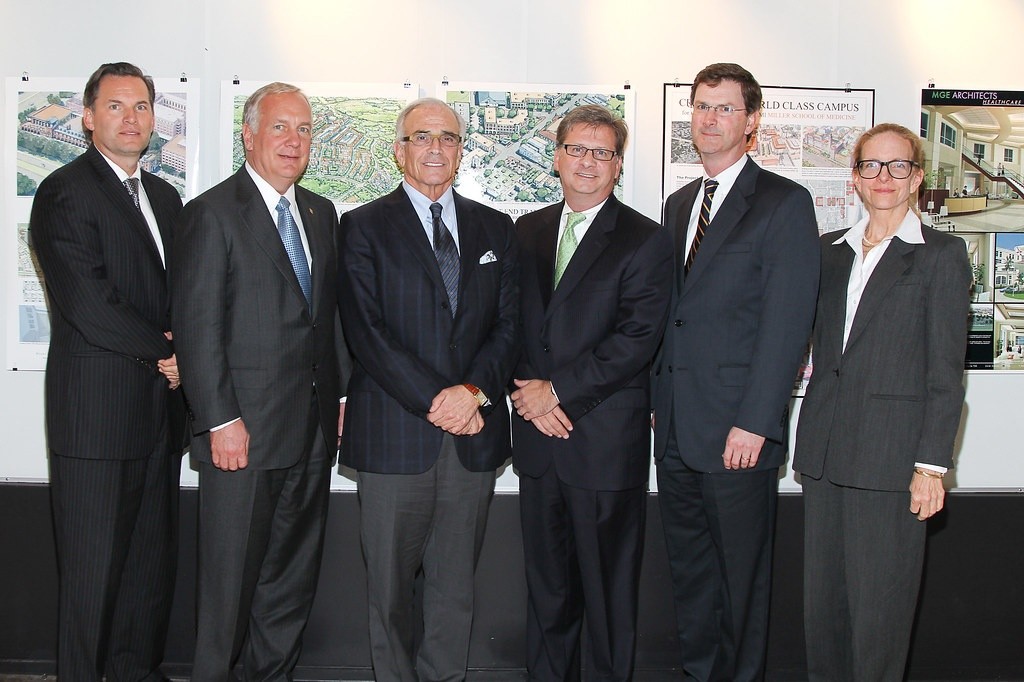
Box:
[932,220,956,232]
[930,213,940,223]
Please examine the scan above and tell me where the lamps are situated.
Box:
[927,200,935,214]
[940,206,948,224]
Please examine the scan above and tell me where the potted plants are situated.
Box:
[971,263,986,294]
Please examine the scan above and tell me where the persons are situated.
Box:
[975,186,980,195]
[961,186,968,196]
[952,188,960,198]
[792,123,973,682]
[171,82,352,682]
[338,99,522,682]
[997,163,1001,176]
[648,63,821,682]
[977,152,981,165]
[508,106,674,682]
[29,62,191,682]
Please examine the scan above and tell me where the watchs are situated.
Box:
[463,384,487,406]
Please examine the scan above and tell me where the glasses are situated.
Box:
[556,144,618,161]
[690,103,750,116]
[854,159,921,179]
[400,133,463,147]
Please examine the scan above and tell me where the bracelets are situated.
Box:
[916,470,942,479]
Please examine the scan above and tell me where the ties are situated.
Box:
[683,178,719,283]
[553,212,586,291]
[275,196,311,307]
[123,178,142,213]
[429,203,461,319]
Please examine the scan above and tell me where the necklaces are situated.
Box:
[864,236,879,245]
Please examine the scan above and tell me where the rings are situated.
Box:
[742,458,750,460]
